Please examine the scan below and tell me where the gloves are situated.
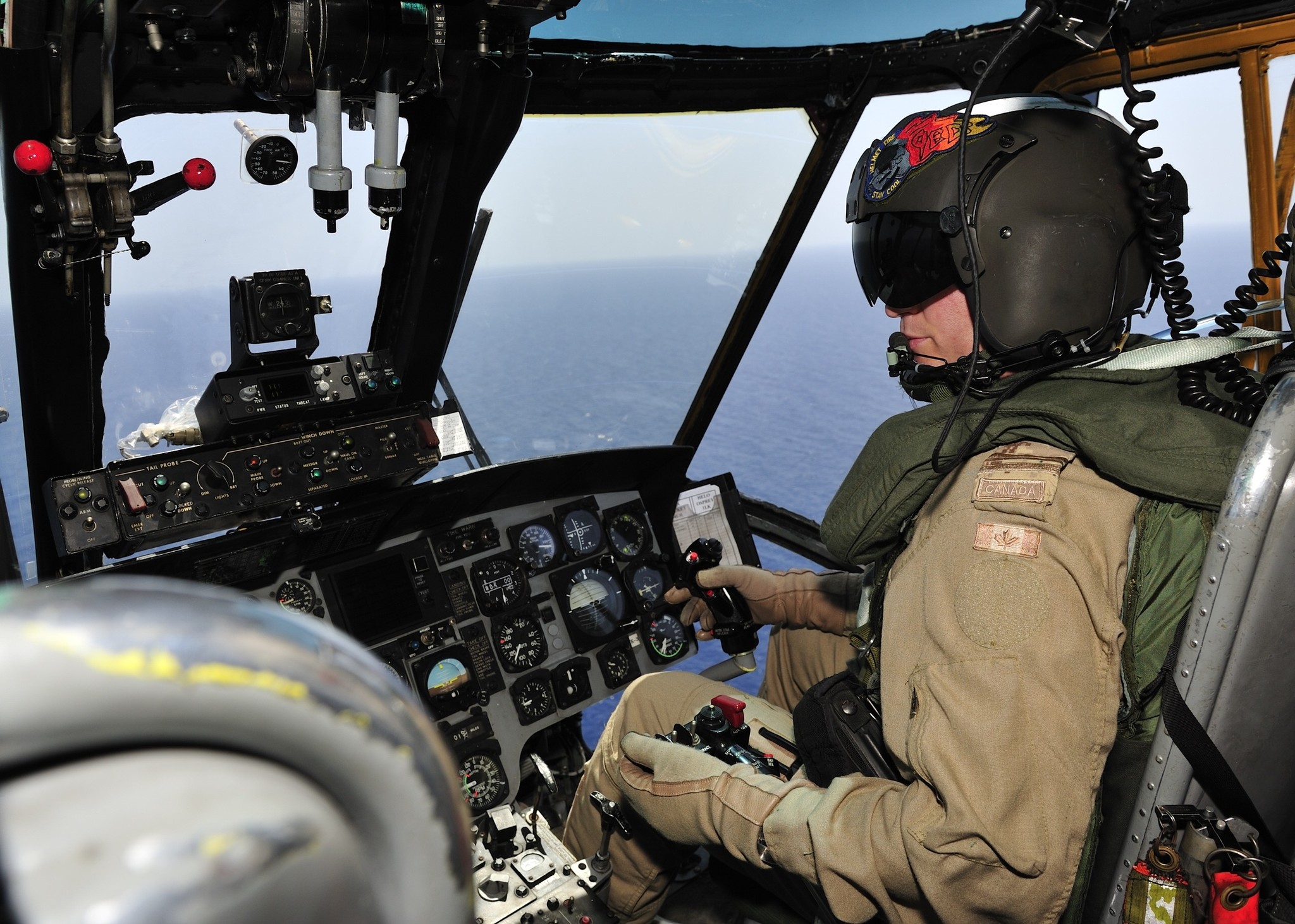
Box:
[616,729,818,875]
[664,561,865,643]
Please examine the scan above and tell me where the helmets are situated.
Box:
[845,88,1191,403]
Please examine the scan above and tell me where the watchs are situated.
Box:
[757,825,778,867]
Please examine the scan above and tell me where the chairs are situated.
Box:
[654,369,1294,924]
[0,574,481,924]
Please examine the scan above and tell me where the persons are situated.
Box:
[573,109,1267,924]
